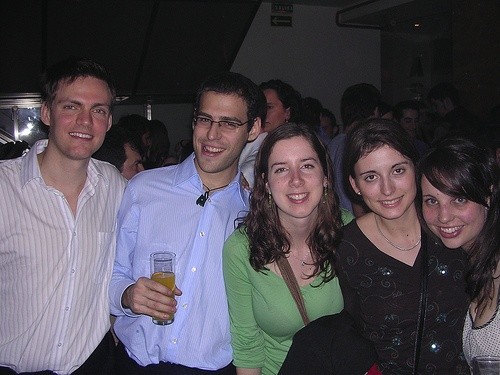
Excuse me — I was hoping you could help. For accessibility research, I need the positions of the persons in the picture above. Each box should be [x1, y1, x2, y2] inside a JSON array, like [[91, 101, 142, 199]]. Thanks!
[[0, 58, 128, 375], [331, 119, 475, 375], [260, 80, 301, 132], [222, 121, 356, 375], [107, 72, 268, 375], [415, 136, 500, 375], [92, 113, 193, 181], [300, 83, 500, 160]]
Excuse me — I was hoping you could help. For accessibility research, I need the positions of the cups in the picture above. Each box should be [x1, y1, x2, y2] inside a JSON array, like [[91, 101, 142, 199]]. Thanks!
[[473, 355, 500, 375], [150, 251, 176, 325]]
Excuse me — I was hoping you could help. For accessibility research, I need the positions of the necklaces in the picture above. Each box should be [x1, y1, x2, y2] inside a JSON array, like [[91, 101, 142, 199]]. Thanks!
[[282, 241, 310, 266], [196, 183, 228, 208], [375, 215, 421, 251]]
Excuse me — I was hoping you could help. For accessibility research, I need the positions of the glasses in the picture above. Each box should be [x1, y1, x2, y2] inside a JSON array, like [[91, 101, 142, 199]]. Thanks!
[[193, 116, 249, 132]]
